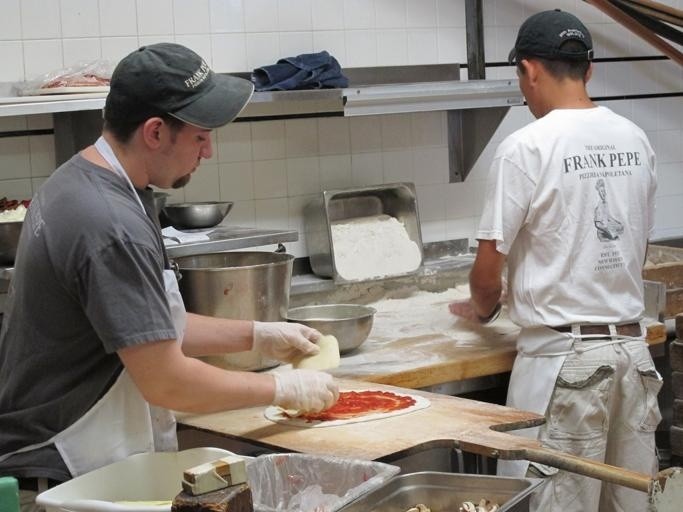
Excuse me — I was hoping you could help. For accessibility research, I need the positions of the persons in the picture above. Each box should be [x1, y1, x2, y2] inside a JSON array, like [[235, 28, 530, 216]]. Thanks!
[[447, 8, 666, 510], [0, 42, 340, 495]]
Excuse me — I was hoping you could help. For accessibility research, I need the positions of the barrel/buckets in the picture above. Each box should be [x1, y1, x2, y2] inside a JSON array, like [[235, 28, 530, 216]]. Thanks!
[[167, 242, 294, 372]]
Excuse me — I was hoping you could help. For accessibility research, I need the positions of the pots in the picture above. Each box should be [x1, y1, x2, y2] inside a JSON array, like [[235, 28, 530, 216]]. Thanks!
[[164, 243, 296, 375]]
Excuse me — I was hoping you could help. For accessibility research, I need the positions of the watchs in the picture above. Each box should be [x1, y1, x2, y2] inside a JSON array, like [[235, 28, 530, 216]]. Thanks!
[[475, 303, 503, 324]]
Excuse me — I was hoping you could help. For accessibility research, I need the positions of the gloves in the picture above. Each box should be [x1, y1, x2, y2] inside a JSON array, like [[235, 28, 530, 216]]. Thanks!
[[255, 320, 321, 361], [272, 370, 339, 412]]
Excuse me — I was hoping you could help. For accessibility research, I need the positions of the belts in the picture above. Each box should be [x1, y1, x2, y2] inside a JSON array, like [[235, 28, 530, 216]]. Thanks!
[[549, 322, 643, 339]]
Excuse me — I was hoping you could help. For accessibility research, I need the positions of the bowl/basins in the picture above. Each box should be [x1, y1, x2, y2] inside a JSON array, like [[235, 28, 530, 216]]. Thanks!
[[288, 304, 376, 355], [335, 470, 546, 512], [161, 201, 233, 227], [34, 446, 254, 511], [247, 453, 401, 512], [307, 185, 427, 280]]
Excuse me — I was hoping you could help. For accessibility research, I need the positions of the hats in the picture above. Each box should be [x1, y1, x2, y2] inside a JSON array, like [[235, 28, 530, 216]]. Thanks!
[[509, 8, 594, 61], [104, 43, 253, 130]]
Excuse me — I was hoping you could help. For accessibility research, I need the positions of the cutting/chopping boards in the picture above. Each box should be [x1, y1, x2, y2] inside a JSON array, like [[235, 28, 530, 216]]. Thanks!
[[330, 293, 664, 391], [174, 378, 652, 493]]
